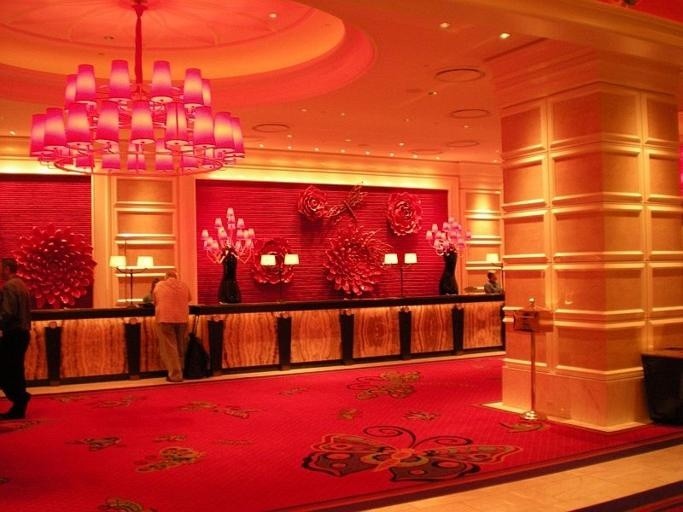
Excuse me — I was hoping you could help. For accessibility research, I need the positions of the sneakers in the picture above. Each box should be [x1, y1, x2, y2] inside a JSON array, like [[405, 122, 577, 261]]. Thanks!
[[0, 391, 31, 420]]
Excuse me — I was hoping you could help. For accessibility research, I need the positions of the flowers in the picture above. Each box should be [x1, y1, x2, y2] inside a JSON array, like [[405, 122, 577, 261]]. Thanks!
[[250, 183, 424, 297], [11, 223, 98, 309]]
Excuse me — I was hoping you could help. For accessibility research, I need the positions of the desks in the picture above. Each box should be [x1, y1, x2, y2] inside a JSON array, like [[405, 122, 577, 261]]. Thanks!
[[1, 299, 512, 384]]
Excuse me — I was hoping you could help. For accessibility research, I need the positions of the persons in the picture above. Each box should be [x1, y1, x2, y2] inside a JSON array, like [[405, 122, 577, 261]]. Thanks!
[[483, 270, 502, 294], [151, 270, 192, 384], [0, 257, 32, 420], [143, 280, 156, 302]]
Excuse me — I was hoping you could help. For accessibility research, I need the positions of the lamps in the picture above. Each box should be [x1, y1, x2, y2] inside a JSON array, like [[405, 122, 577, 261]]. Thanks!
[[110, 249, 156, 304], [28, 0, 248, 176], [201, 210, 302, 305]]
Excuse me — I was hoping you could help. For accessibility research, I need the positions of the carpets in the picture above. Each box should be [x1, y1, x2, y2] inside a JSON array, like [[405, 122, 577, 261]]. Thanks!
[[1, 347, 682, 512]]
